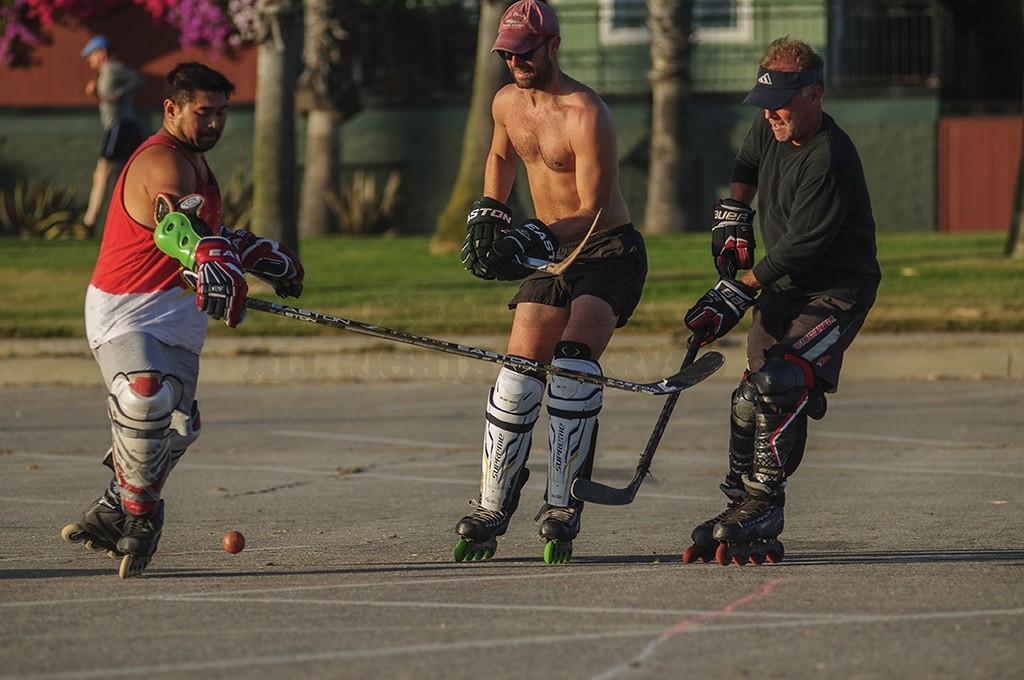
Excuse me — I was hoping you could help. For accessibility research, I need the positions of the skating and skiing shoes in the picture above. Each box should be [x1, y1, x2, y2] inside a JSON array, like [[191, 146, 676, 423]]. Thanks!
[[679, 477, 748, 563], [533, 503, 581, 563], [60, 489, 133, 560], [451, 496, 511, 560], [714, 472, 788, 568], [116, 498, 167, 579]]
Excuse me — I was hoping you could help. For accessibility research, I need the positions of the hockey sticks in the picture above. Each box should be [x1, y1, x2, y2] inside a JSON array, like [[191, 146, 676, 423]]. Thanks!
[[571, 329, 709, 506], [510, 208, 604, 276], [179, 267, 726, 396]]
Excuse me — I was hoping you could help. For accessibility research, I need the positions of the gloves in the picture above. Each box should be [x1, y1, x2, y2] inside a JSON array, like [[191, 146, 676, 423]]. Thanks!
[[222, 227, 302, 298], [192, 237, 248, 326], [492, 218, 556, 280], [460, 197, 512, 280], [712, 198, 756, 278], [685, 275, 757, 349]]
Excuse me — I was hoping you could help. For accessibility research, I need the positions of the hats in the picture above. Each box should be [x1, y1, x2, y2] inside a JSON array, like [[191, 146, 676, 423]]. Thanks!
[[493, 0, 558, 55], [742, 59, 821, 112], [80, 36, 106, 58]]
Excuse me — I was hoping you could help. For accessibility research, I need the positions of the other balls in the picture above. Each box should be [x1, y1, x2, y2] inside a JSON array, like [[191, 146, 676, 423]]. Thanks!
[[223, 531, 245, 554]]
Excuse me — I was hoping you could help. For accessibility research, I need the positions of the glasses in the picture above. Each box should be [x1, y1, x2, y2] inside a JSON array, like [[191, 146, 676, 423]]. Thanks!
[[496, 42, 550, 62]]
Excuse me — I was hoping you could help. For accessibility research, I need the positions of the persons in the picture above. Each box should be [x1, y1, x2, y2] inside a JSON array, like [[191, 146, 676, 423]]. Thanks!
[[684, 39, 882, 544], [80, 36, 143, 226], [80, 58, 306, 552], [456, 0, 648, 539]]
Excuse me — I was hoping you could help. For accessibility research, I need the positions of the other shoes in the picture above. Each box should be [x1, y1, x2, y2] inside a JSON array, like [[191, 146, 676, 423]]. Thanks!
[[61, 225, 93, 239]]
